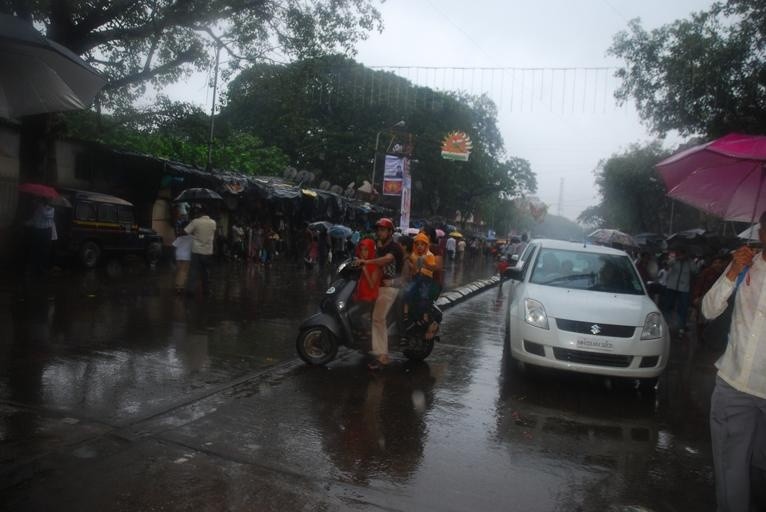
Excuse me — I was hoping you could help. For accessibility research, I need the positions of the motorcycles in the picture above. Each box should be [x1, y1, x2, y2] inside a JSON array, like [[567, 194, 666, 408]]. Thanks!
[[296, 240, 442, 366]]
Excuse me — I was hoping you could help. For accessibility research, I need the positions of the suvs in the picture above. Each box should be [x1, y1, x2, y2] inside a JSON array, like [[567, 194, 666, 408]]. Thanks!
[[54, 187, 164, 271]]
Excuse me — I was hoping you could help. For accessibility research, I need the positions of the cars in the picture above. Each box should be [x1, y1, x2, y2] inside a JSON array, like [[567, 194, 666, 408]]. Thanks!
[[504, 238, 670, 396]]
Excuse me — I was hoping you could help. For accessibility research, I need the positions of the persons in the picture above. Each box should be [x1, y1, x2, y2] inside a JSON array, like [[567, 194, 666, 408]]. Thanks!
[[396, 166, 403, 178], [624, 245, 738, 341], [701, 213, 766, 511], [168, 208, 280, 294], [352, 217, 438, 370], [305, 216, 527, 261], [27, 200, 58, 275]]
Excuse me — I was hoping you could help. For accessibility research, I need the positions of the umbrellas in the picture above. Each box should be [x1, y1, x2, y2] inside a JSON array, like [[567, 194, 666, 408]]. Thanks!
[[17, 180, 62, 201], [654, 133, 766, 247], [588, 223, 760, 252]]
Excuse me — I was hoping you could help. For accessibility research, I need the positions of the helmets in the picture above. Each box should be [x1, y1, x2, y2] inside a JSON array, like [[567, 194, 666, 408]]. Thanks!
[[376, 218, 393, 229]]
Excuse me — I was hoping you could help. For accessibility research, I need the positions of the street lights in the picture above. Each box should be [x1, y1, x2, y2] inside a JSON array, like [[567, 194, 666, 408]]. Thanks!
[[206, 62, 218, 164], [371, 121, 406, 188]]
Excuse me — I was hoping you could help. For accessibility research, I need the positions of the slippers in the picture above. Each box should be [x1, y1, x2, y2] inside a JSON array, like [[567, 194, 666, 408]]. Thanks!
[[426, 322, 439, 339], [370, 360, 393, 370]]
